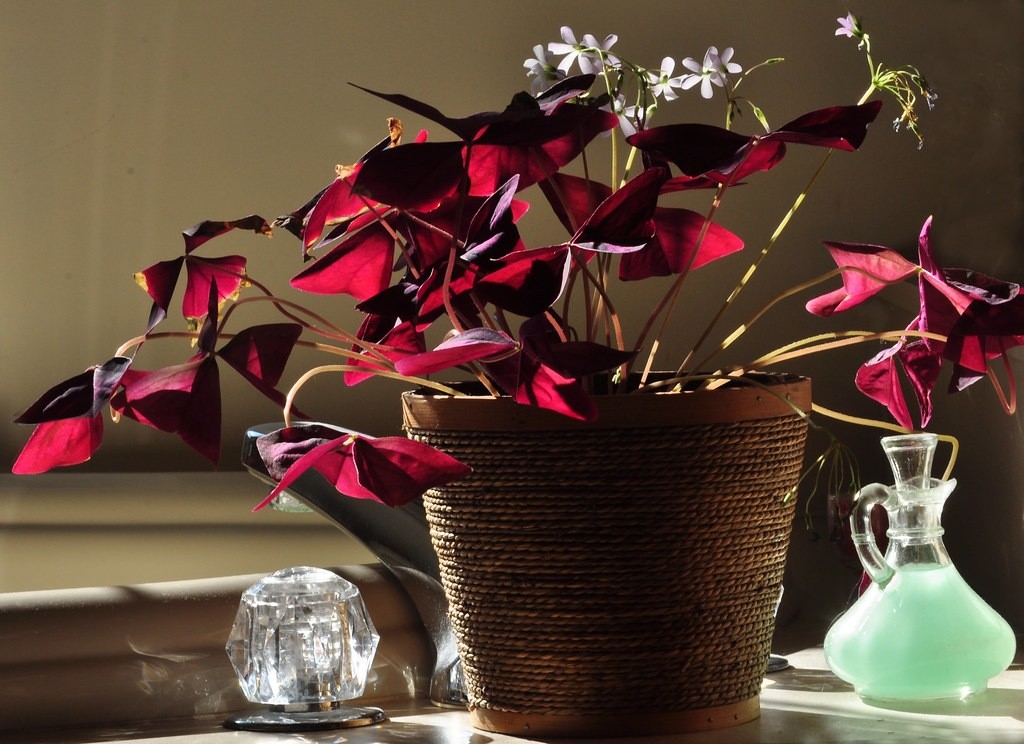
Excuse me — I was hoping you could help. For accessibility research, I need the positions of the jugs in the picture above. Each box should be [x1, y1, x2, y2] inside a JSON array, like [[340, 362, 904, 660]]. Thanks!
[[821, 433, 1017, 701]]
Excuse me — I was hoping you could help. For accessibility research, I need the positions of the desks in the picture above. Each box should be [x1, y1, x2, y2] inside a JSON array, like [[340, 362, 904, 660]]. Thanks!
[[83, 642, 1024, 744]]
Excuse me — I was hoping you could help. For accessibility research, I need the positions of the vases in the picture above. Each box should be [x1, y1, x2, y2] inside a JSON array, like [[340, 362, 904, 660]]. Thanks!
[[826, 431, 1015, 708]]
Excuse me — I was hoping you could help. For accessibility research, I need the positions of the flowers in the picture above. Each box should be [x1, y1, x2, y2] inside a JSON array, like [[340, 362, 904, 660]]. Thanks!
[[398, 369, 810, 737], [14, 10, 1023, 515]]
